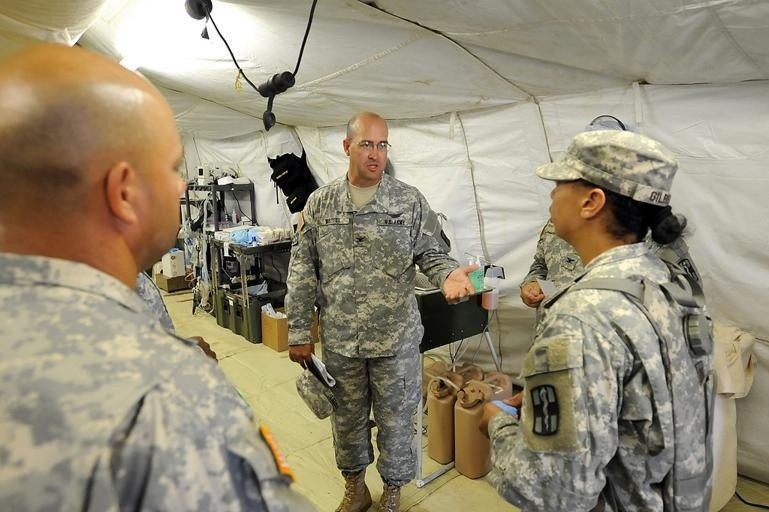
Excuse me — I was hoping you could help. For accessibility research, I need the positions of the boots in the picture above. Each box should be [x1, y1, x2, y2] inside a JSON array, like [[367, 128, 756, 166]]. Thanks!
[[335, 469, 372, 512], [377, 483, 401, 512]]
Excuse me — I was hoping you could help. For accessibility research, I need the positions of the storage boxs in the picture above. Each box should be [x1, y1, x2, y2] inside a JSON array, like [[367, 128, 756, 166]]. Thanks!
[[155, 273, 188, 292]]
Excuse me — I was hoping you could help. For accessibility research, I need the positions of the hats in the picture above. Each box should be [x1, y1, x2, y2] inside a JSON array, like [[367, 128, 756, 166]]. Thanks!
[[297, 358, 339, 419], [536, 129, 678, 207]]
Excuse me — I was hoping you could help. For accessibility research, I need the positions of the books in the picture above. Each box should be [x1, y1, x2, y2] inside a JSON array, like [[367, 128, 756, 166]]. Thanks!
[[304, 350, 336, 388]]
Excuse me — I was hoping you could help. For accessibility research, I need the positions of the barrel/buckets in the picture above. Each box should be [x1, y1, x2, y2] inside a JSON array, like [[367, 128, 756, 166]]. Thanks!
[[427, 362, 485, 465], [454, 373, 514, 480]]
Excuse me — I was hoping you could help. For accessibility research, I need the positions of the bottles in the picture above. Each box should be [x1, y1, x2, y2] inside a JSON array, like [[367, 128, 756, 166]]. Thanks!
[[198, 169, 205, 186]]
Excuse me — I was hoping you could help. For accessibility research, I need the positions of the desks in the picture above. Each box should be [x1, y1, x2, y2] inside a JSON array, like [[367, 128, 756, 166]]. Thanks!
[[198, 239, 292, 344]]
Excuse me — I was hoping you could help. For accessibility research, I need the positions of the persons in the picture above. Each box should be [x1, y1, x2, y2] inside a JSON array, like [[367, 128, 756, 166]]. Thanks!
[[476, 130, 714, 511], [284, 112, 480, 512], [520, 122, 701, 330], [134, 265, 220, 363], [0, 43, 318, 512]]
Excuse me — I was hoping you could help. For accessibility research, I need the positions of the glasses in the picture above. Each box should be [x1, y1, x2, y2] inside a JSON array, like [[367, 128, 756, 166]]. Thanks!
[[360, 142, 391, 152]]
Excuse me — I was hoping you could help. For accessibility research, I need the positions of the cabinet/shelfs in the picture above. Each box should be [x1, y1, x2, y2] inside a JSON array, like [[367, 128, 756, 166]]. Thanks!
[[185, 185, 255, 318]]
